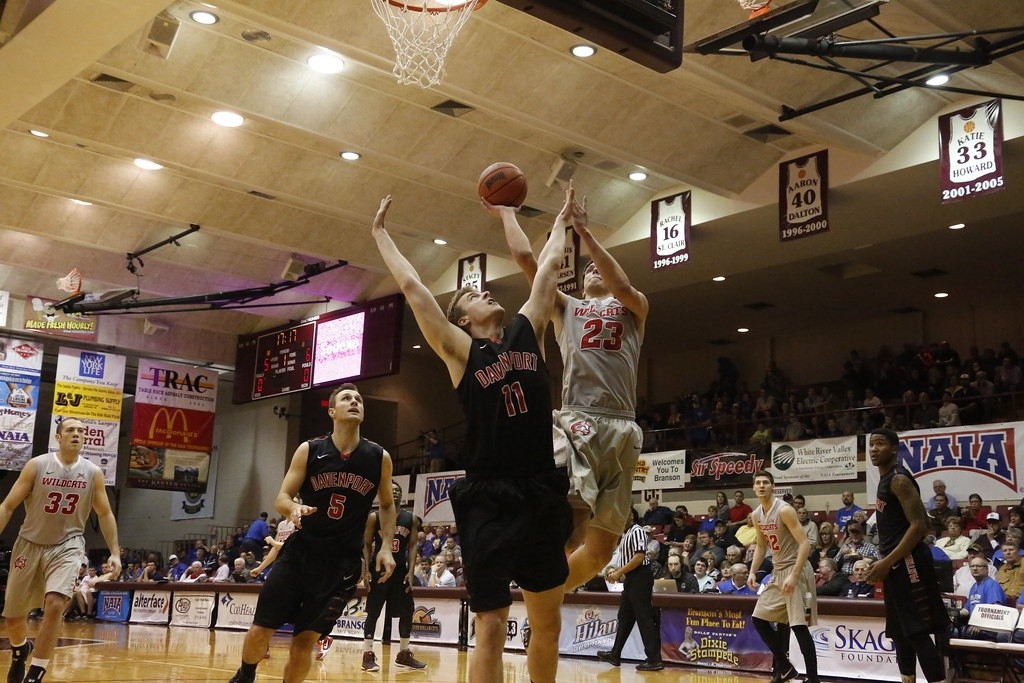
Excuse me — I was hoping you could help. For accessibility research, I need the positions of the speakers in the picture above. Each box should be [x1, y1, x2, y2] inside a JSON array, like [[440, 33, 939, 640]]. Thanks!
[[545, 156, 579, 191]]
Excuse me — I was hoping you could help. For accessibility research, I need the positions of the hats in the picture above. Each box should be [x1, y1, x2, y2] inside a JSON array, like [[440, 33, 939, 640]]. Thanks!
[[1006, 528, 1024, 540], [643, 525, 653, 533], [169, 554, 178, 560], [261, 512, 268, 517], [783, 494, 793, 499], [986, 512, 1001, 520], [715, 519, 726, 524], [966, 544, 984, 551], [247, 551, 255, 557], [850, 523, 862, 532]]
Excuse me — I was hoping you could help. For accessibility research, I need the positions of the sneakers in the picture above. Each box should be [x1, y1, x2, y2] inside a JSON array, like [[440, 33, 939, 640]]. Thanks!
[[23, 665, 46, 683], [7, 640, 34, 683], [769, 666, 799, 683], [803, 677, 819, 683], [265, 644, 270, 657], [361, 651, 380, 671], [316, 635, 334, 659], [395, 648, 427, 669], [636, 660, 662, 670], [597, 651, 621, 666]]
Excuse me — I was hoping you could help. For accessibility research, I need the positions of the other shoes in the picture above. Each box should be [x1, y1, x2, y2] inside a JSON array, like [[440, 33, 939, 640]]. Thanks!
[[229, 668, 255, 683]]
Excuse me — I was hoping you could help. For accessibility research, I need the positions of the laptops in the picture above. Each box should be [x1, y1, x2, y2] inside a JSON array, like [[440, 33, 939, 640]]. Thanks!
[[653, 579, 678, 593]]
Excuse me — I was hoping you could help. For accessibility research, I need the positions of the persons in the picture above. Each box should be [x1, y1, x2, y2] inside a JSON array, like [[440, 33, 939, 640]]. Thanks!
[[362, 482, 428, 672], [0, 416, 122, 683], [356, 522, 466, 588], [249, 497, 333, 660], [424, 429, 445, 473], [635, 340, 1023, 460], [479, 195, 649, 595], [229, 383, 397, 683], [597, 508, 664, 672], [744, 469, 820, 683], [370, 178, 577, 683], [65, 517, 277, 620], [572, 480, 1024, 644], [861, 428, 952, 683]]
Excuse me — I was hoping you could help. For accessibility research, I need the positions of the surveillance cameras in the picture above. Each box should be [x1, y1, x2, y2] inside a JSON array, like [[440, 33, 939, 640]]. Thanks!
[[51, 292, 87, 310]]
